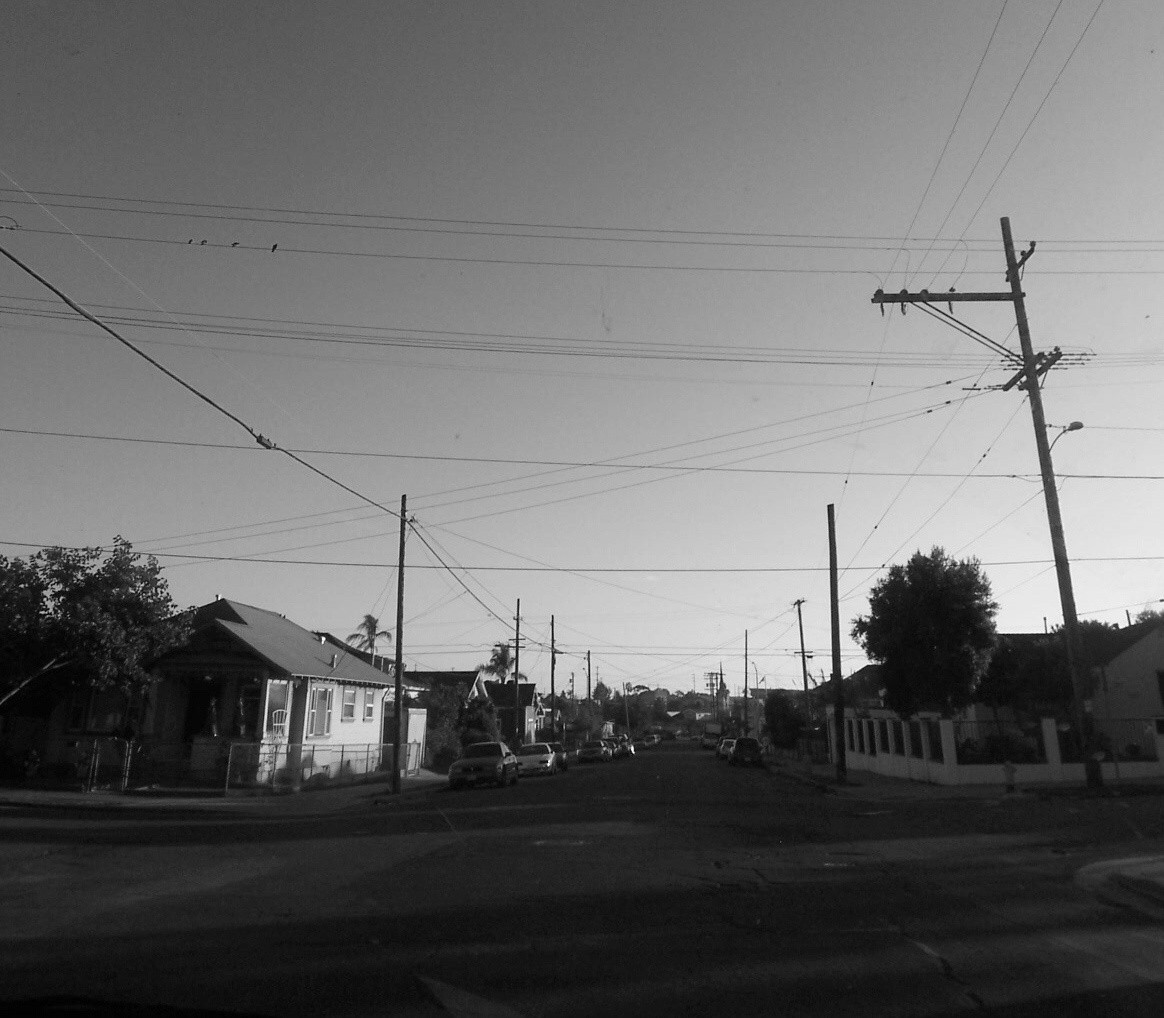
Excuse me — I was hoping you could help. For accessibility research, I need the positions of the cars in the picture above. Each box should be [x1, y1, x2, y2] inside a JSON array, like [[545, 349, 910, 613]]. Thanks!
[[727, 738, 765, 767], [448, 742, 520, 791], [577, 734, 637, 763], [515, 743, 560, 777], [633, 732, 663, 750], [546, 741, 569, 772], [700, 733, 736, 760]]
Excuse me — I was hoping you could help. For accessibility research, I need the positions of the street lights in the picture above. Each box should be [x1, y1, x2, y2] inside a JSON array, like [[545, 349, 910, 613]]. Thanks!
[[1039, 421, 1110, 797]]
